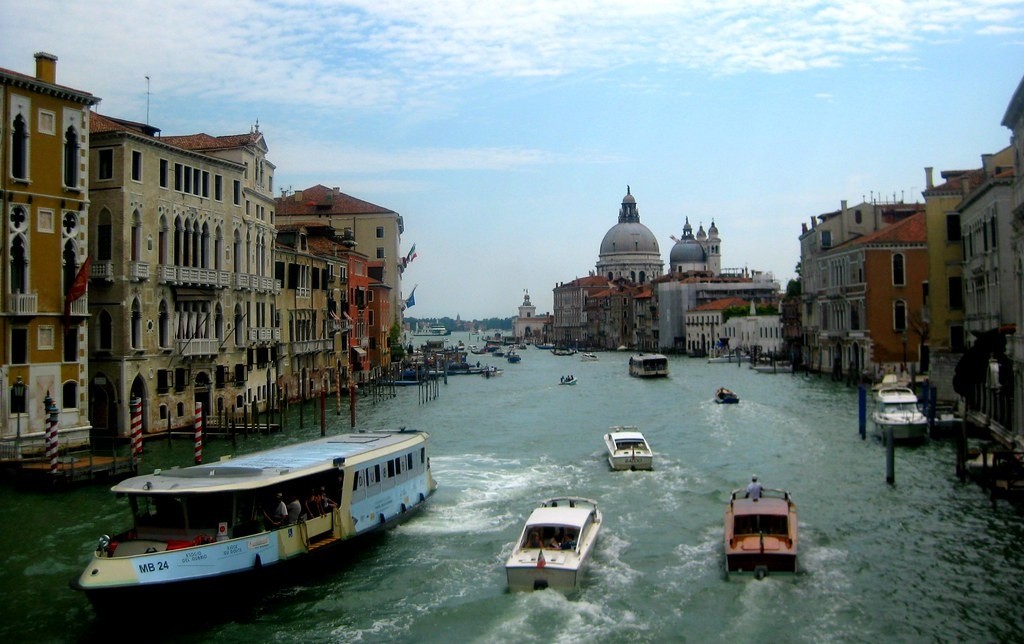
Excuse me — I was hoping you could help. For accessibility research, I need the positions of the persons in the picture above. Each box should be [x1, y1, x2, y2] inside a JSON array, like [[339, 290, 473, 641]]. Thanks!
[[476, 361, 480, 367], [264, 484, 338, 525], [879, 362, 905, 372], [561, 375, 574, 382], [526, 527, 576, 549], [744, 475, 763, 498], [718, 388, 726, 400], [491, 366, 494, 371], [403, 357, 417, 370]]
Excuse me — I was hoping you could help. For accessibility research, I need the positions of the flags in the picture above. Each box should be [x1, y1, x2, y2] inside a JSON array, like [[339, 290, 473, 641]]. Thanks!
[[538, 552, 546, 572], [406, 244, 417, 261], [65, 257, 89, 303], [406, 286, 415, 308]]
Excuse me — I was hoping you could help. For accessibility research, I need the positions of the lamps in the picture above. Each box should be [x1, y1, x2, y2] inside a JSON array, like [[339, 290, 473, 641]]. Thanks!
[[153, 467, 161, 476], [143, 482, 152, 490], [332, 456, 346, 467]]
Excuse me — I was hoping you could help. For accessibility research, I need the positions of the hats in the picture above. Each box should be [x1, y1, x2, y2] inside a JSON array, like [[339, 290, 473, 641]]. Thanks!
[[276, 493, 283, 498]]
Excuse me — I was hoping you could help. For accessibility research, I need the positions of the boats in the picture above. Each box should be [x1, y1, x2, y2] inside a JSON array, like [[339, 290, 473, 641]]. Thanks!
[[603, 424, 654, 472], [627, 352, 671, 379], [67, 425, 440, 632], [752, 364, 793, 374], [707, 353, 751, 363], [714, 386, 740, 405], [580, 352, 598, 362], [558, 374, 577, 385], [617, 345, 628, 352], [724, 486, 800, 581], [399, 323, 579, 376], [504, 493, 603, 596], [870, 383, 928, 447]]
